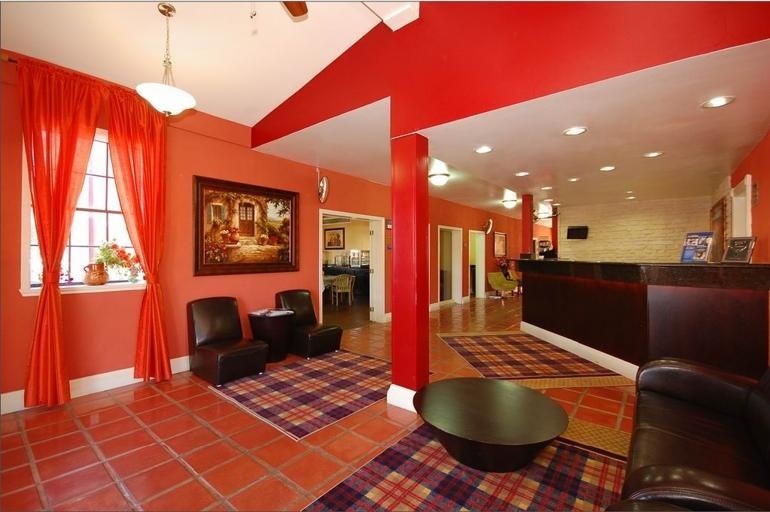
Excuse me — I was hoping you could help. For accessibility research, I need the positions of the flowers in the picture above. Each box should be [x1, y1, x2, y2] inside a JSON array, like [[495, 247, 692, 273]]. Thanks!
[[93, 240, 147, 277]]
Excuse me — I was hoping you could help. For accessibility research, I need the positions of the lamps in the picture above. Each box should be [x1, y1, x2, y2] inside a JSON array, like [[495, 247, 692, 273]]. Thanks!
[[502, 190, 517, 209], [482, 218, 494, 235], [429, 162, 452, 186], [316, 168, 329, 204], [133, 2, 196, 117]]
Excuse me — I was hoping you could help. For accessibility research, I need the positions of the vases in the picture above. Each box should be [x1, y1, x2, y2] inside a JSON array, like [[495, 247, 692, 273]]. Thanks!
[[127, 273, 144, 284], [82, 262, 109, 288]]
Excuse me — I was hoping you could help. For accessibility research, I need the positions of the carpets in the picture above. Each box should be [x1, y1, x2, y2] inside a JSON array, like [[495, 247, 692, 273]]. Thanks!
[[436, 330, 635, 388], [299, 416, 627, 512], [208, 346, 437, 443]]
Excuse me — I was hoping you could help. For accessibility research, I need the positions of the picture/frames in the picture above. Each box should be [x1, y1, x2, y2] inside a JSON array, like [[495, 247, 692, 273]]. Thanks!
[[681, 231, 714, 262], [721, 237, 756, 264], [191, 174, 300, 277], [493, 232, 508, 258], [324, 227, 345, 250]]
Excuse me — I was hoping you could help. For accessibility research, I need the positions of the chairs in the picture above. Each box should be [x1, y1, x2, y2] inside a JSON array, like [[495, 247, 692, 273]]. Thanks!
[[330, 273, 355, 307], [488, 271, 515, 299]]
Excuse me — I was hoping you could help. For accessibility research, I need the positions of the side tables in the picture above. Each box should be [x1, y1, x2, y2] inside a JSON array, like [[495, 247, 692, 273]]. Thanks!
[[248, 307, 294, 360]]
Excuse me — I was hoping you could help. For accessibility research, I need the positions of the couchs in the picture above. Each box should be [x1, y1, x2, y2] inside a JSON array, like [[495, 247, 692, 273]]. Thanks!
[[274, 288, 343, 361], [187, 296, 268, 388], [604, 355, 770, 512]]
